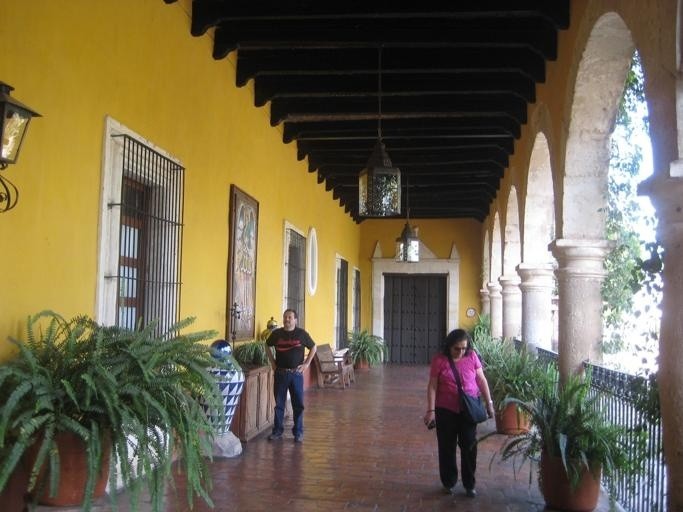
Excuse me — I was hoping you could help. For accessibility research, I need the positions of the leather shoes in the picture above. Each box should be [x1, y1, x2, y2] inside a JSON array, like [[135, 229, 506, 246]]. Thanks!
[[269, 434, 281, 440], [444, 486, 454, 494], [466, 489, 476, 497], [294, 436, 303, 441]]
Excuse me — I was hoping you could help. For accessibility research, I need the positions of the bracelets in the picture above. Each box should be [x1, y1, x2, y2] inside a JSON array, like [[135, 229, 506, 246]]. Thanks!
[[427, 409, 435, 414], [487, 400, 493, 408]]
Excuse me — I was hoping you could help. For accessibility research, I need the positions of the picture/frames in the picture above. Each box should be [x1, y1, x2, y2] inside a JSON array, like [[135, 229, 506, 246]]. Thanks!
[[225, 184, 256, 342]]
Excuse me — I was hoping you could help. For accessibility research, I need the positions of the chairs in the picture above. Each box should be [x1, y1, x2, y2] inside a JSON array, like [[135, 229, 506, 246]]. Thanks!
[[313, 343, 356, 389]]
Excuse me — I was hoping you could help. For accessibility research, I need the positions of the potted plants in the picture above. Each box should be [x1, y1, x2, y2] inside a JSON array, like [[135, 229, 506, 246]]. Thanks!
[[488, 362, 650, 511], [484, 352, 560, 434], [347, 326, 390, 369], [0, 310, 224, 511]]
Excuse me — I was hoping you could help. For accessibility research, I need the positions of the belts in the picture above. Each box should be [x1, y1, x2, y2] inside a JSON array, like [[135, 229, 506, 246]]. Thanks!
[[279, 368, 295, 372]]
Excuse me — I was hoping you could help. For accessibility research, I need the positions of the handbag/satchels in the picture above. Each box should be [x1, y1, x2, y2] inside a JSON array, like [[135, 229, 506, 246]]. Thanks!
[[461, 395, 491, 423]]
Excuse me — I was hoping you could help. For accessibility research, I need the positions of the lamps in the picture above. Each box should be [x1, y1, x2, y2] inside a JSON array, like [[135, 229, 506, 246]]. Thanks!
[[397, 167, 422, 263], [0, 83, 41, 211], [359, 47, 401, 217]]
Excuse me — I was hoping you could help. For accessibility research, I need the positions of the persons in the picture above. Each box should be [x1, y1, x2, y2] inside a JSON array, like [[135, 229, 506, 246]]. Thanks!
[[423, 329, 496, 497], [265, 309, 317, 443]]
